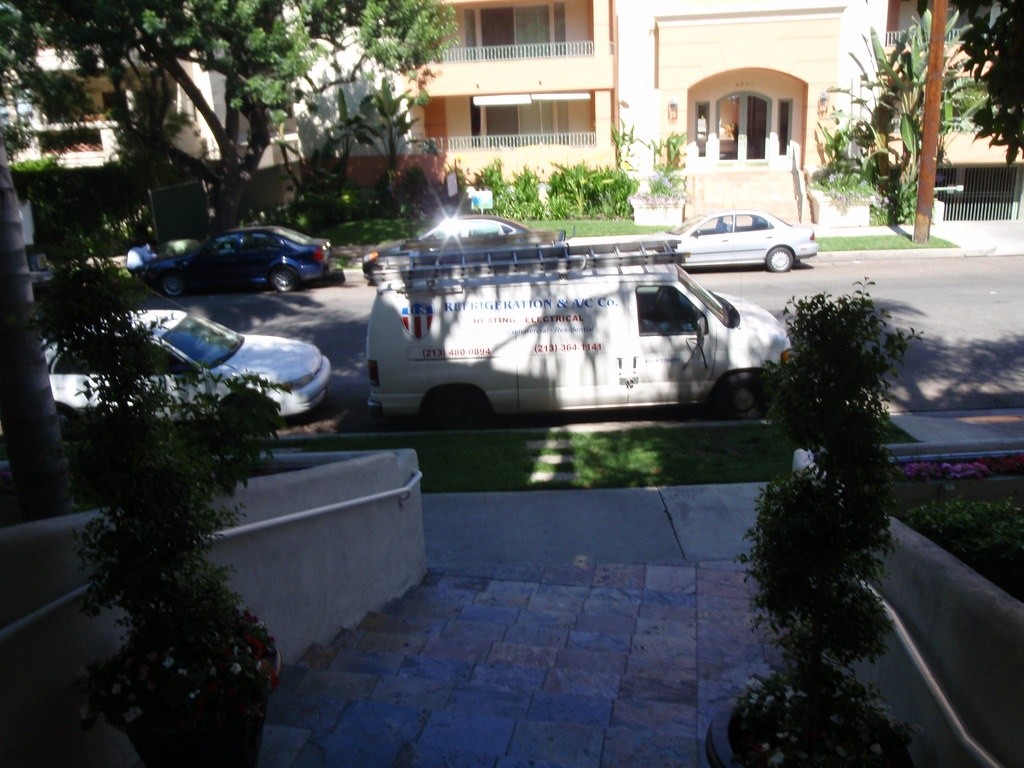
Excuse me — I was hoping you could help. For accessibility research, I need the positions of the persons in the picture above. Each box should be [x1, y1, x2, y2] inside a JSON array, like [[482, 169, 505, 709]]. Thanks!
[[127, 240, 161, 276]]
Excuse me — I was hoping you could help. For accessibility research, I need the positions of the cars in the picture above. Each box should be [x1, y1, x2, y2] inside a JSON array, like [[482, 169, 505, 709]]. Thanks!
[[652, 208, 821, 273], [362, 213, 556, 285], [40, 306, 334, 417], [135, 225, 332, 300]]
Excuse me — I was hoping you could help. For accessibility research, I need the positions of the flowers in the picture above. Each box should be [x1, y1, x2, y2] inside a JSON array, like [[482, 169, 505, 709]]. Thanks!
[[80, 608, 281, 732], [737, 674, 924, 768]]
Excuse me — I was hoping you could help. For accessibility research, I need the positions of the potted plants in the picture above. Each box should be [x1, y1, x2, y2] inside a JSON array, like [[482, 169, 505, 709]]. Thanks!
[[29, 214, 291, 768], [704, 277, 926, 768]]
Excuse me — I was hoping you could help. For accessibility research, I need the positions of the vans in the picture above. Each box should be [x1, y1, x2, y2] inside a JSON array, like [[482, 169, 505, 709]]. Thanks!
[[364, 235, 792, 428]]
